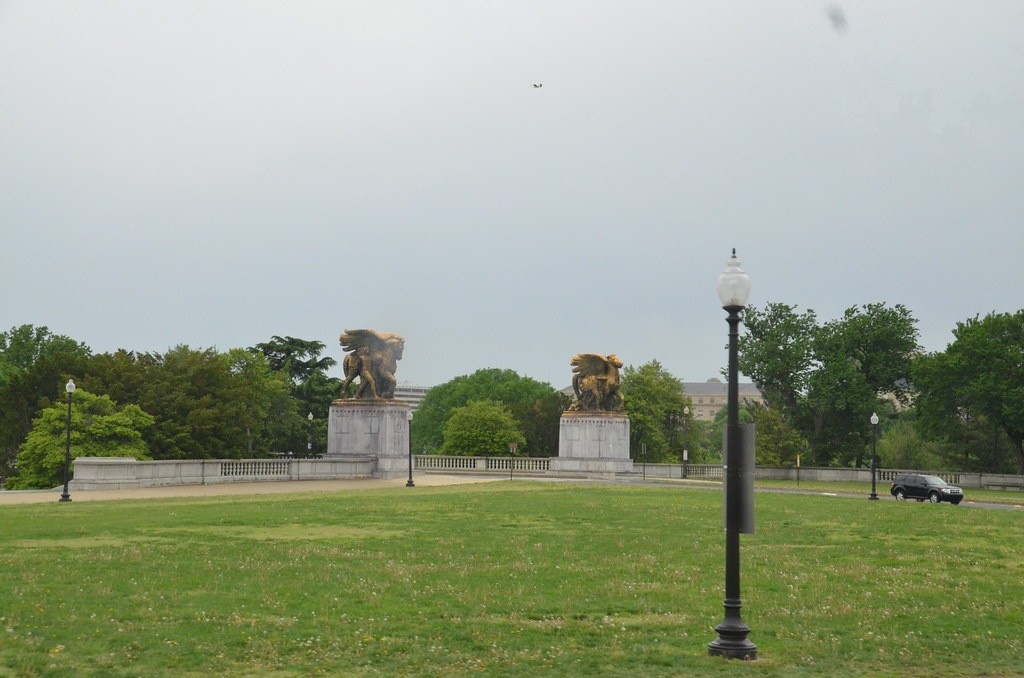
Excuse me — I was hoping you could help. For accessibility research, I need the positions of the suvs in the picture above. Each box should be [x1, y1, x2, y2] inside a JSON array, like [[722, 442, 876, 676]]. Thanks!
[[890, 473, 964, 505]]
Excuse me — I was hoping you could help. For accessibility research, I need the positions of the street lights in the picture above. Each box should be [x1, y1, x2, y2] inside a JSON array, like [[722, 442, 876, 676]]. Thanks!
[[406, 408, 415, 487], [307, 412, 313, 458], [868, 412, 880, 501], [711, 245, 760, 662], [681, 406, 690, 479], [57, 377, 76, 502]]
